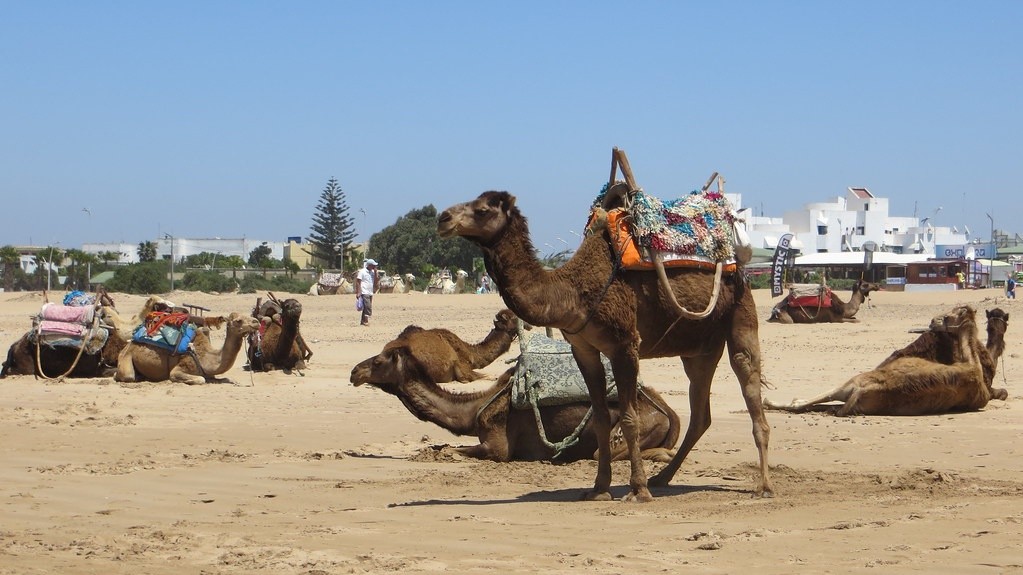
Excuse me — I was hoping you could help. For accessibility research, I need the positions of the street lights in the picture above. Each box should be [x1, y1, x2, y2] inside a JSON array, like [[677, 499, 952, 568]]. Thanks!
[[986, 213, 994, 287], [47, 241, 61, 292], [164, 232, 174, 291]]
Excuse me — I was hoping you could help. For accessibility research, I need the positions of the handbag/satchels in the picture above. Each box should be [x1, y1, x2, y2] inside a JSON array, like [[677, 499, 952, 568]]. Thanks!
[[357, 296, 363, 311]]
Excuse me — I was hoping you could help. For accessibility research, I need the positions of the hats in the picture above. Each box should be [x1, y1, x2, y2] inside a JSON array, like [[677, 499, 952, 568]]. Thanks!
[[367, 259, 378, 265]]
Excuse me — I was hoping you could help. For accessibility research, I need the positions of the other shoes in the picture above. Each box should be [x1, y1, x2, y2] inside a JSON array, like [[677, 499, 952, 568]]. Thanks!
[[362, 320, 369, 326]]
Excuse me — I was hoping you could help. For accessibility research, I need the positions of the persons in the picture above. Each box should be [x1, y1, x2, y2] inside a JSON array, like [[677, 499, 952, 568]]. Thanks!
[[357, 258, 379, 327], [1006, 274, 1015, 299], [955, 268, 964, 289], [785, 268, 809, 285], [477, 271, 492, 294]]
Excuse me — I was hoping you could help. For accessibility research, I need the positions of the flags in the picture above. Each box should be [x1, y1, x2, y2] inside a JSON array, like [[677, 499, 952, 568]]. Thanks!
[[771, 234, 793, 298]]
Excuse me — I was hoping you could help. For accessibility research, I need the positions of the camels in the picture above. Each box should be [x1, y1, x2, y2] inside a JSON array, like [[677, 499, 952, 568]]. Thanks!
[[245, 296, 304, 373], [876, 309, 1009, 400], [439, 180, 773, 501], [764, 306, 990, 417], [767, 279, 879, 322], [351, 310, 682, 462], [0, 305, 144, 378], [112, 297, 261, 385]]
